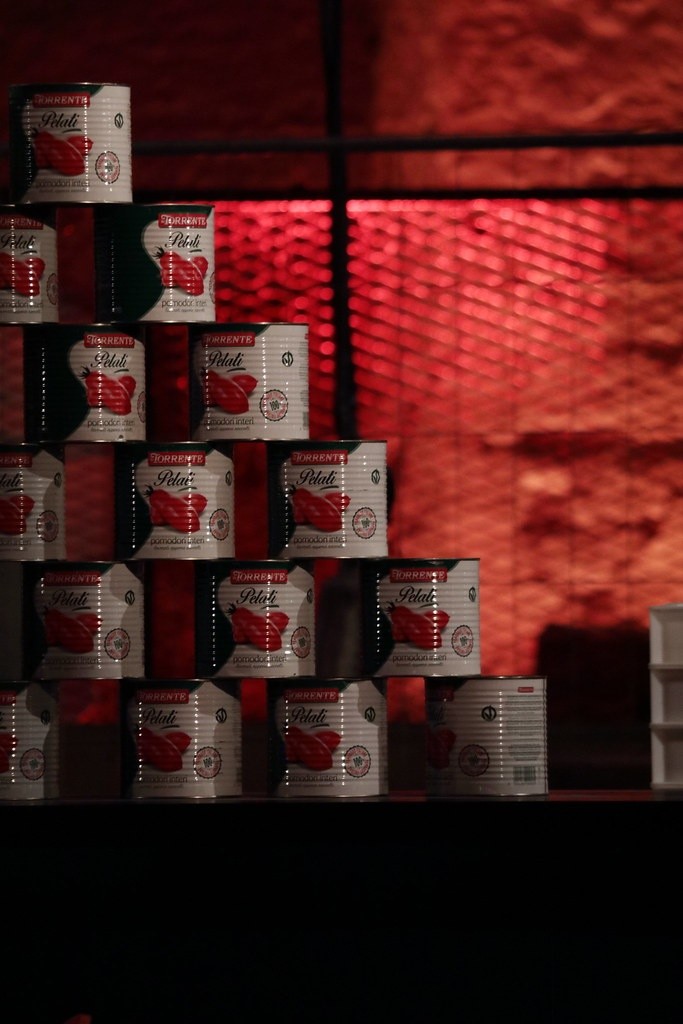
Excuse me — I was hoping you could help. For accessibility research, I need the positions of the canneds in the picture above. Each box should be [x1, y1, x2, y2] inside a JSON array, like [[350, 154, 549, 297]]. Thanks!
[[0, 322, 548, 806], [0, 80, 216, 324]]
